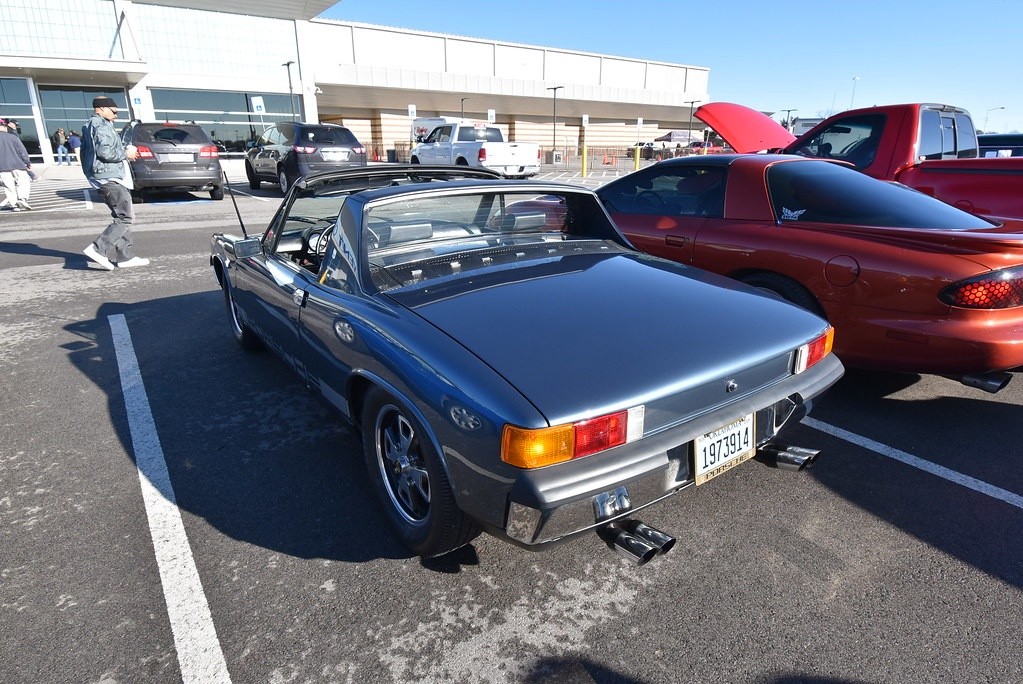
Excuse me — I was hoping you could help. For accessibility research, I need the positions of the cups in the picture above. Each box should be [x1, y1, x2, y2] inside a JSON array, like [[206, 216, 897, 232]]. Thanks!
[[126, 145, 137, 160]]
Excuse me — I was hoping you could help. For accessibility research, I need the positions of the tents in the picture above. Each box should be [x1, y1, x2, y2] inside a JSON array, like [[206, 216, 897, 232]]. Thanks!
[[652, 131, 700, 162]]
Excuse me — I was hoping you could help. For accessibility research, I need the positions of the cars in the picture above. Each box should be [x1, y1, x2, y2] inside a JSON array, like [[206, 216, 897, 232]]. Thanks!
[[491, 156, 1023, 395], [208, 166, 845, 565]]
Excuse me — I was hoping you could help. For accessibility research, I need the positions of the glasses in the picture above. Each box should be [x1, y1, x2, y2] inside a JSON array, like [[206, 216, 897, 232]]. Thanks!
[[107, 106, 117, 115]]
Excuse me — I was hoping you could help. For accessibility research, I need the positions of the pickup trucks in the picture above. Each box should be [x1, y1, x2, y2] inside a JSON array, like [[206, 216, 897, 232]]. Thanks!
[[409, 121, 542, 182], [683, 142, 725, 154], [690, 102, 1023, 236]]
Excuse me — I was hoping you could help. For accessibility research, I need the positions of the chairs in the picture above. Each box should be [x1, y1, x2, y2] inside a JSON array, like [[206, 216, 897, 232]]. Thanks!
[[502, 211, 546, 233], [379, 222, 433, 245]]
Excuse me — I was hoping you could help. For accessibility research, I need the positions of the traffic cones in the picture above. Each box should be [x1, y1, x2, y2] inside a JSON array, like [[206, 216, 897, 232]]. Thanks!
[[602, 154, 608, 165]]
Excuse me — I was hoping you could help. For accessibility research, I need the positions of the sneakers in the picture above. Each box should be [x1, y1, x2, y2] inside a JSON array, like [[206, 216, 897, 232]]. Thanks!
[[83, 243, 114, 271], [117, 256, 150, 267]]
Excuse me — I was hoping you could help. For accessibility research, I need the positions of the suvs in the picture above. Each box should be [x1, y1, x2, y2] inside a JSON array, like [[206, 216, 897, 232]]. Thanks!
[[244, 121, 367, 196], [627, 141, 659, 157], [120, 119, 226, 202]]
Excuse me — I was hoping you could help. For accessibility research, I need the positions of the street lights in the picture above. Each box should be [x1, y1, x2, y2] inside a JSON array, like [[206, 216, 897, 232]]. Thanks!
[[547, 86, 565, 150], [282, 60, 296, 122], [780, 108, 797, 128], [461, 97, 468, 118], [851, 76, 860, 106]]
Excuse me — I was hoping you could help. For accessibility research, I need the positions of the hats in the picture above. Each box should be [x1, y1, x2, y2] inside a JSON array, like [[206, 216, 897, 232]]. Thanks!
[[10, 119, 20, 126]]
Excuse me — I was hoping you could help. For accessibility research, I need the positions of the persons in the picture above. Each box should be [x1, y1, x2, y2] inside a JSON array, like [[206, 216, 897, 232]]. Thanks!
[[81, 95, 150, 271], [52, 127, 83, 166], [0, 118, 34, 212]]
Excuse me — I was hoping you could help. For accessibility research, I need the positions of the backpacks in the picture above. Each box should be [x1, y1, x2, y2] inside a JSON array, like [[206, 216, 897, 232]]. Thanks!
[[80, 120, 103, 190]]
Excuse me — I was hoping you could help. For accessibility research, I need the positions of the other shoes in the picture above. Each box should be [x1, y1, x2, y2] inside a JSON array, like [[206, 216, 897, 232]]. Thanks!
[[68, 162, 72, 166], [58, 163, 61, 165], [13, 207, 21, 211], [18, 199, 32, 210]]
